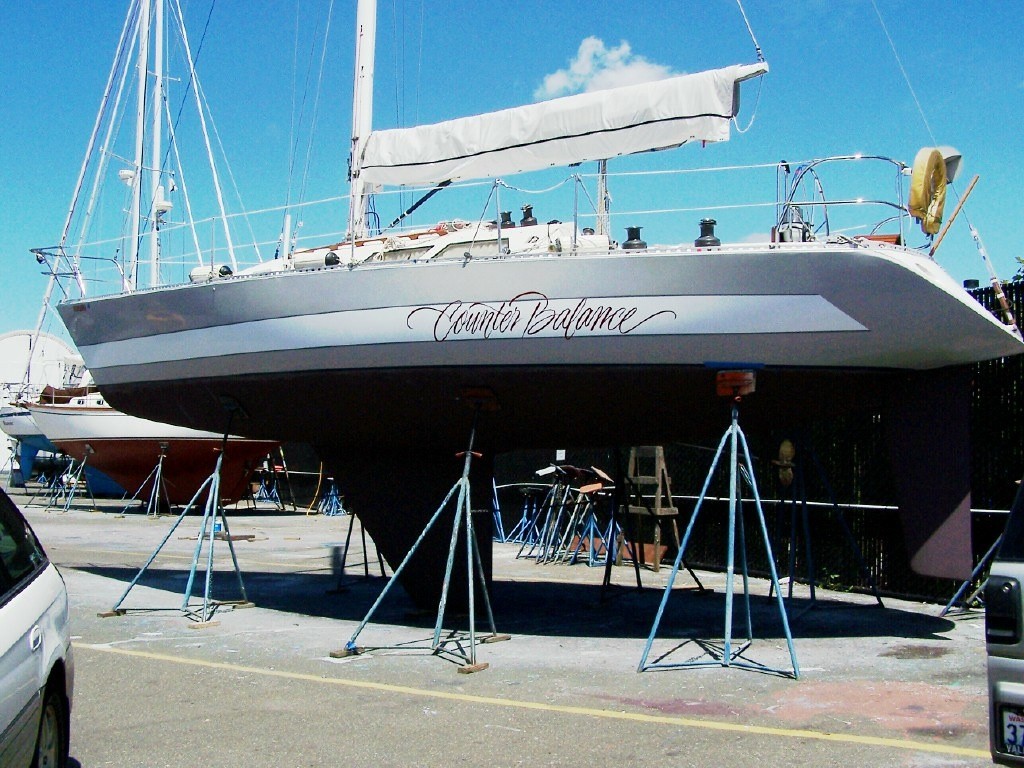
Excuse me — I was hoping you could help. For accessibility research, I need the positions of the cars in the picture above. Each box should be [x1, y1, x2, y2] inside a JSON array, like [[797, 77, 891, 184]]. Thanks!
[[980, 475, 1024, 768], [0, 484, 75, 768]]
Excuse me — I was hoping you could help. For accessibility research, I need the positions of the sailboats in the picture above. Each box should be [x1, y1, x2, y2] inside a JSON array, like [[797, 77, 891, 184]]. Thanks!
[[0, 2, 1024, 609]]
